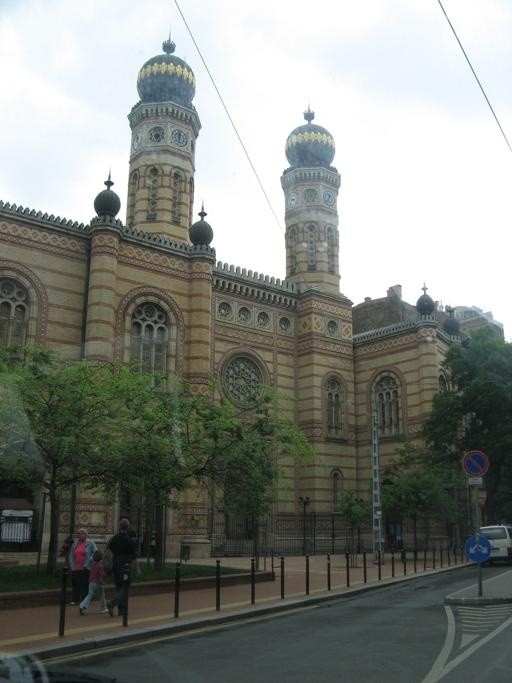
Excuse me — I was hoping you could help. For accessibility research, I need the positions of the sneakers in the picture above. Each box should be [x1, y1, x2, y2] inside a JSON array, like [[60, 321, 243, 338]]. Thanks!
[[70, 600, 121, 617]]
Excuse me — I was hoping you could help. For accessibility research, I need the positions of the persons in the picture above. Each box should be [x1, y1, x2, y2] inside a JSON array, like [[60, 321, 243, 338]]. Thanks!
[[104, 517, 137, 615], [69, 527, 97, 604], [78, 549, 109, 616]]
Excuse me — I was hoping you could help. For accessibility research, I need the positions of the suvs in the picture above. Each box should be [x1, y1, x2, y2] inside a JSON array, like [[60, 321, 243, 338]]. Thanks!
[[479, 522, 512, 567]]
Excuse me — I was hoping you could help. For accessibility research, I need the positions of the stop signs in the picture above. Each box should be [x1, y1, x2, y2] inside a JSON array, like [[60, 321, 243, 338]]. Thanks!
[[463, 450, 489, 477]]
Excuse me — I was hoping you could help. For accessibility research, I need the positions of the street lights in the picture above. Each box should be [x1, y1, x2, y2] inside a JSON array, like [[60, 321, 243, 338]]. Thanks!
[[298, 495, 311, 556]]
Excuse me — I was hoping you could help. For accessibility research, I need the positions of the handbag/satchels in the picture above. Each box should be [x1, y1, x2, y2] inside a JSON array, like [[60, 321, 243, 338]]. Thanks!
[[103, 550, 113, 569]]
